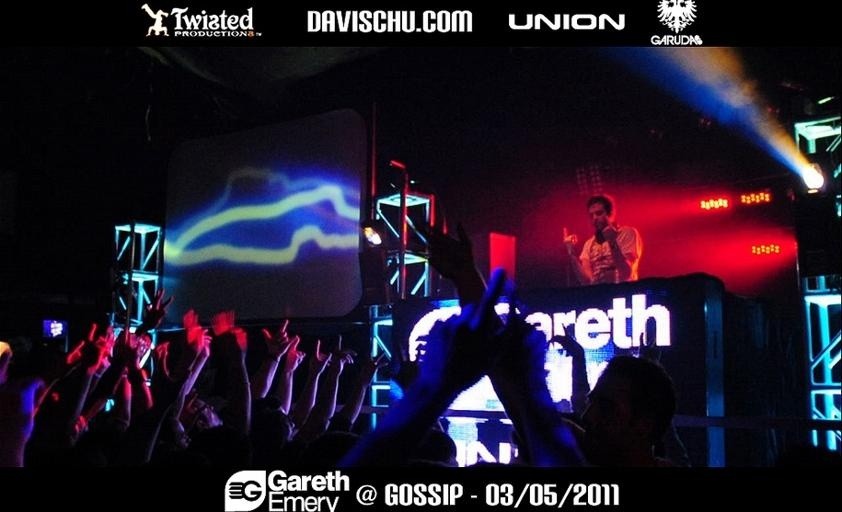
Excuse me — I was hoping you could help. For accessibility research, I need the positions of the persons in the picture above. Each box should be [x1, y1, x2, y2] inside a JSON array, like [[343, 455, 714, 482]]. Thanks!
[[562, 191, 643, 286]]
